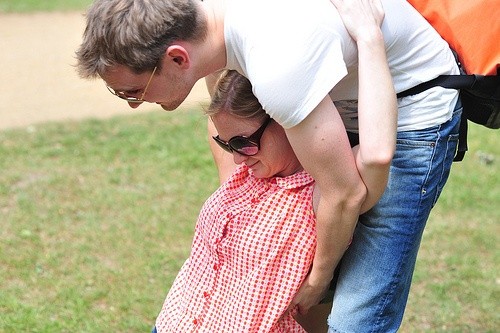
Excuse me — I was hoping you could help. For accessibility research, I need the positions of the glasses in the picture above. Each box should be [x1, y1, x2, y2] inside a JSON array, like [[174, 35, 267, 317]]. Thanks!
[[105, 64, 159, 103], [212, 116, 274, 157]]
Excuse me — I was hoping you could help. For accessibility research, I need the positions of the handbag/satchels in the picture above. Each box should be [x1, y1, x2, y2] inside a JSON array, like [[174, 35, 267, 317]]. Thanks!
[[406, 1, 500, 131]]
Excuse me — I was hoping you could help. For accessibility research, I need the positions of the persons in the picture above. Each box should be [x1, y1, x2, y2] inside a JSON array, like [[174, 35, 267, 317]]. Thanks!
[[76, 0, 465, 332], [149, 0, 400, 332]]
